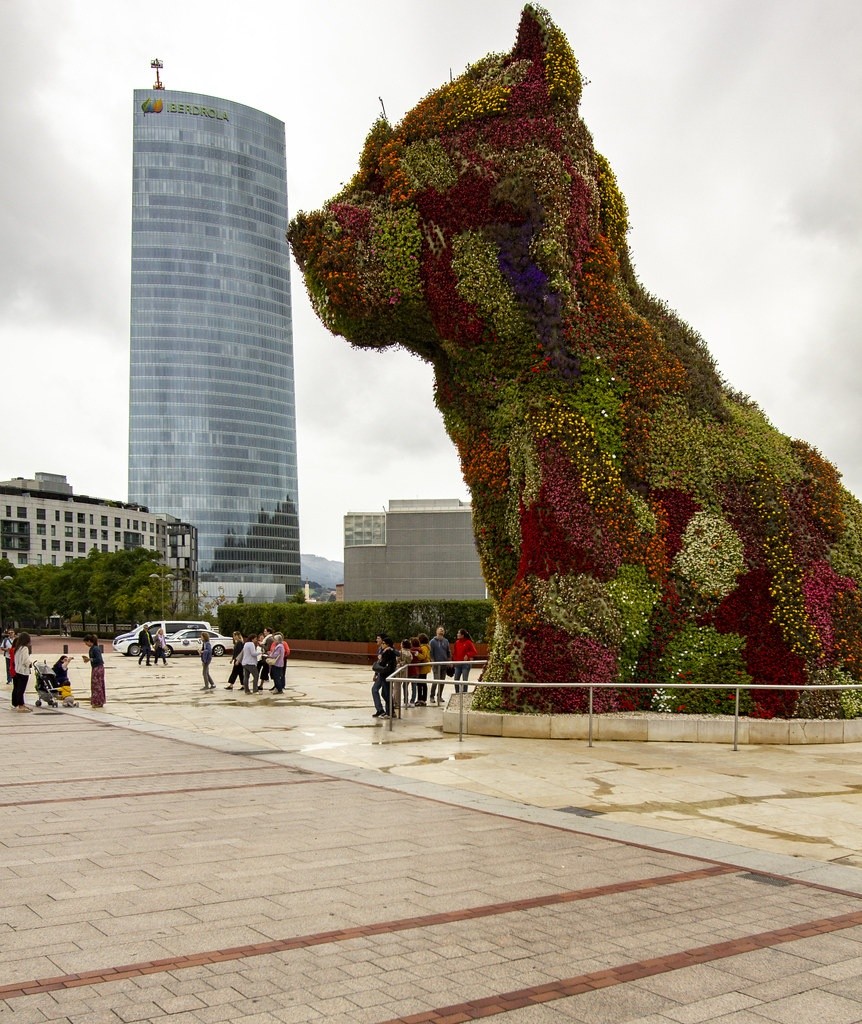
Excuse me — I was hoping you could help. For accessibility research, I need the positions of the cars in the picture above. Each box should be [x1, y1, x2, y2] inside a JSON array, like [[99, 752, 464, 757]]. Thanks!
[[149, 627, 238, 660]]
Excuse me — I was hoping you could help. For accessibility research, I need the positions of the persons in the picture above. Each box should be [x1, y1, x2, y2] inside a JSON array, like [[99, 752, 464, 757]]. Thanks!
[[9, 633, 33, 712], [371, 633, 432, 719], [199, 632, 217, 690], [57, 680, 79, 708], [52, 655, 73, 698], [138, 625, 154, 666], [82, 635, 106, 708], [451, 630, 478, 693], [1, 629, 17, 684], [223, 628, 290, 695], [152, 628, 169, 666], [430, 627, 451, 703]]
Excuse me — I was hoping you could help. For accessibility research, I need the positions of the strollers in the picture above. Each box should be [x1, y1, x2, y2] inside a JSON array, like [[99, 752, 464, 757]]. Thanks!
[[31, 659, 61, 709]]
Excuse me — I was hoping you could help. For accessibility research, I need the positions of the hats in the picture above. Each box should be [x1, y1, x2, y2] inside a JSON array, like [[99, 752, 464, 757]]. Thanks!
[[274, 632, 284, 640], [274, 635, 283, 643], [377, 633, 393, 646]]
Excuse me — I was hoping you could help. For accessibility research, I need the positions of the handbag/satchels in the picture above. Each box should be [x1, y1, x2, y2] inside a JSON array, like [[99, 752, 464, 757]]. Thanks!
[[265, 658, 276, 665], [447, 667, 455, 677], [165, 646, 168, 651], [372, 661, 385, 673]]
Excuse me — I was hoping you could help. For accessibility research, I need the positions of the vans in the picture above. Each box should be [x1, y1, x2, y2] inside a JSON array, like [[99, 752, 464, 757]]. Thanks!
[[112, 617, 216, 659]]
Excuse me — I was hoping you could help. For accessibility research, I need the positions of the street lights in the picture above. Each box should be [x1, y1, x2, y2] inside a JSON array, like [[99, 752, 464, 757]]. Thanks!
[[149, 558, 179, 621], [0, 576, 13, 644]]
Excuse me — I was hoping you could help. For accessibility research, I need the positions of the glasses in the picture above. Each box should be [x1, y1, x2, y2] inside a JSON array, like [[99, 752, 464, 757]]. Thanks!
[[9, 632, 14, 634], [382, 643, 387, 645]]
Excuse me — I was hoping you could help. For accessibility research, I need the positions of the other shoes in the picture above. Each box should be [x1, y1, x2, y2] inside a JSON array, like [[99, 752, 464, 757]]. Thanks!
[[11, 705, 31, 712], [138, 662, 151, 666], [271, 688, 282, 694], [6, 679, 13, 684], [67, 701, 79, 708], [378, 712, 397, 719], [200, 685, 216, 690], [394, 697, 445, 709], [269, 686, 276, 691], [223, 686, 264, 695], [372, 710, 385, 718], [155, 662, 168, 666]]
